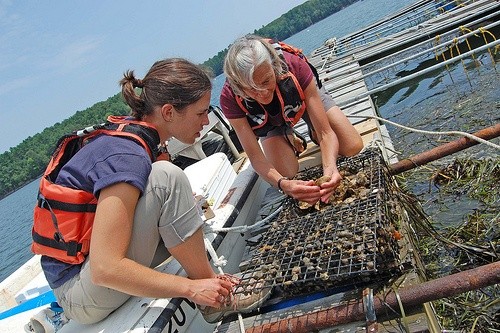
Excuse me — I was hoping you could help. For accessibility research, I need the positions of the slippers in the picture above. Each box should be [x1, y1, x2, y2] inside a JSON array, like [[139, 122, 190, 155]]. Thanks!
[[292, 129, 307, 152], [307, 124, 320, 146]]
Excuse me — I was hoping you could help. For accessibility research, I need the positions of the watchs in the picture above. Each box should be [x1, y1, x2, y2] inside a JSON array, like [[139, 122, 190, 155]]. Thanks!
[[278, 176, 289, 194]]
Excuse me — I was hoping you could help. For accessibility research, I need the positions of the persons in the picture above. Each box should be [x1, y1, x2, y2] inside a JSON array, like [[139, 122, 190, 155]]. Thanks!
[[31, 57, 274, 324], [219, 34, 363, 216]]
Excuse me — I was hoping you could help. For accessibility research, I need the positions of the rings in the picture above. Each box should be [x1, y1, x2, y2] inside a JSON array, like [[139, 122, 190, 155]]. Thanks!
[[220, 296, 224, 303]]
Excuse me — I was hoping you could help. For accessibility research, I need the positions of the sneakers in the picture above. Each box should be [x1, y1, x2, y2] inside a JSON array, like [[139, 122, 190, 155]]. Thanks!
[[196, 286, 273, 324]]
